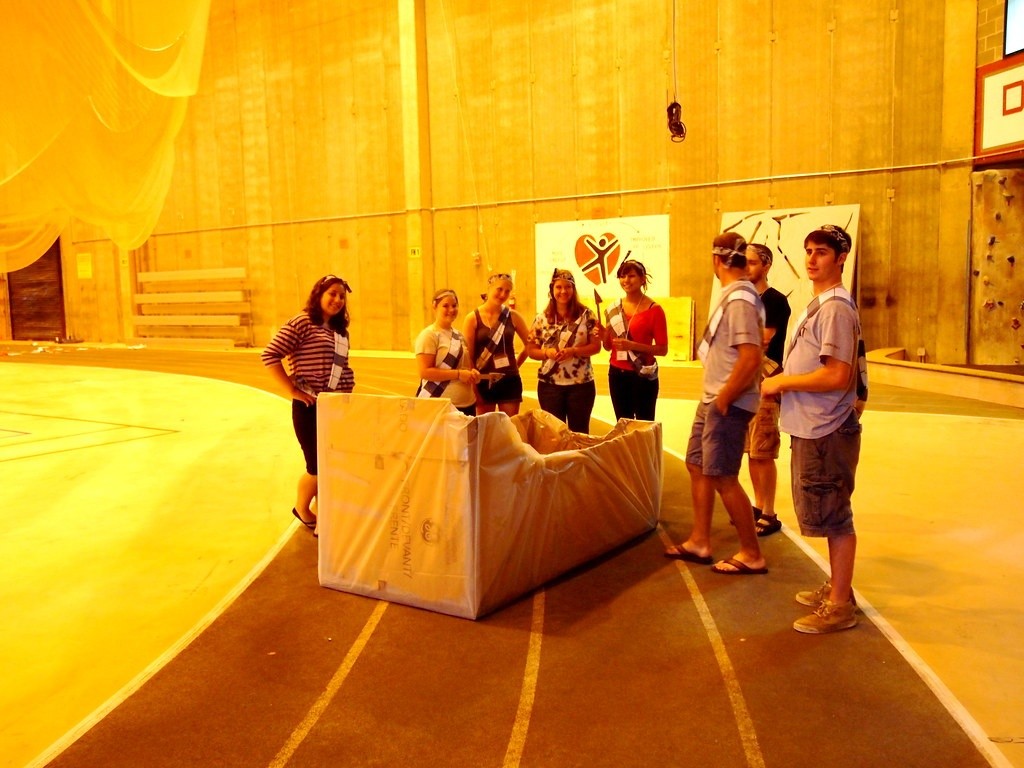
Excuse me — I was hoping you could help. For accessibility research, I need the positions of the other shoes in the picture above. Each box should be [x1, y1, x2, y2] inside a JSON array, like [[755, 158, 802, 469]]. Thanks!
[[795, 581, 856, 607], [794, 599, 857, 633]]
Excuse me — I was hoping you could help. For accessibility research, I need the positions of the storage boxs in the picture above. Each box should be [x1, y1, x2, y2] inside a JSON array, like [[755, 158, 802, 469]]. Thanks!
[[316, 391, 662, 620]]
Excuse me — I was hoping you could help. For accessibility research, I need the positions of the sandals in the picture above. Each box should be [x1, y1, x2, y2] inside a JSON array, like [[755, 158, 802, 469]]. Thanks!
[[730, 507, 764, 523], [756, 514, 781, 536]]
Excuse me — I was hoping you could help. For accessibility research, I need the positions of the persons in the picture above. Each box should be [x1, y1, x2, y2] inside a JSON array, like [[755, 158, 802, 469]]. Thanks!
[[464, 274, 529, 419], [667, 232, 769, 573], [261, 274, 355, 537], [595, 259, 669, 422], [759, 225, 867, 634], [413, 288, 481, 418], [523, 269, 601, 433], [729, 244, 792, 536]]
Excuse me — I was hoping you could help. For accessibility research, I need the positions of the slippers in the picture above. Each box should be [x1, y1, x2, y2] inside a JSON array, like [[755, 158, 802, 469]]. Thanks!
[[292, 507, 319, 539], [664, 543, 713, 565], [711, 555, 769, 574]]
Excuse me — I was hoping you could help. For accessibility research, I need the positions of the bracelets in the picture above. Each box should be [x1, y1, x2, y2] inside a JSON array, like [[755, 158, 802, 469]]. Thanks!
[[457, 369, 460, 380]]
